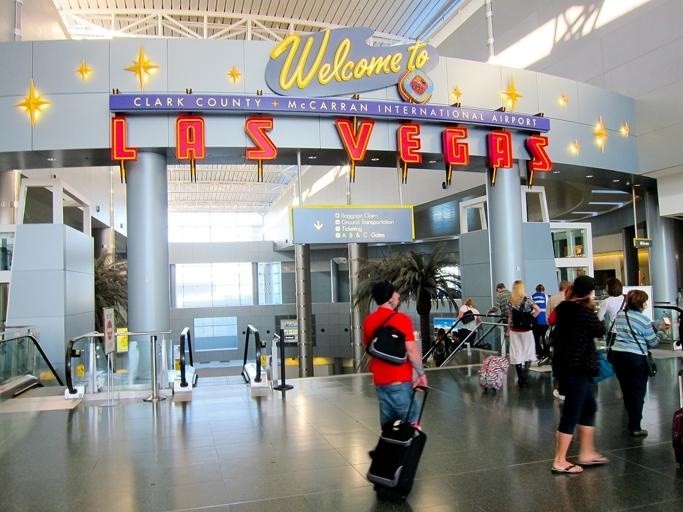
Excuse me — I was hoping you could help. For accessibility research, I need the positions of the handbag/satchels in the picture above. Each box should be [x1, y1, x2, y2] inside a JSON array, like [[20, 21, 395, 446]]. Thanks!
[[606, 332, 616, 347], [511, 309, 536, 330], [461, 310, 474, 324], [590, 352, 614, 385], [365, 327, 408, 366], [643, 351, 657, 377]]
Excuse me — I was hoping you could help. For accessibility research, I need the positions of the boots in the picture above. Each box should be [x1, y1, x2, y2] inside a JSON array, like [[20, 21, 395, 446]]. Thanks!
[[517, 363, 531, 387]]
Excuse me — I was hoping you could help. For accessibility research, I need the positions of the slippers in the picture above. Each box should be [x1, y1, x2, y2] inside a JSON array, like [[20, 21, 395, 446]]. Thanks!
[[551, 464, 582, 474], [577, 455, 610, 464]]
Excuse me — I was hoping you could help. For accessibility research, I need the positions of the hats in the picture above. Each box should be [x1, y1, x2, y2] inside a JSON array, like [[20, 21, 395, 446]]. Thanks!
[[568, 276, 593, 301], [372, 281, 395, 305]]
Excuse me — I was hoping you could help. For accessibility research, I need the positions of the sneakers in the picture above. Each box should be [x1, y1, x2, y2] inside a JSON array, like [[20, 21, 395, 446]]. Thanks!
[[553, 389, 565, 399], [538, 356, 553, 366], [634, 429, 647, 438]]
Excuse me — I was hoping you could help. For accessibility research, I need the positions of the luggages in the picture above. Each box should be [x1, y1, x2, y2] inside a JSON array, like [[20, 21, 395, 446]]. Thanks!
[[672, 369, 683, 469], [365, 386, 429, 503], [476, 327, 491, 350], [478, 335, 509, 396]]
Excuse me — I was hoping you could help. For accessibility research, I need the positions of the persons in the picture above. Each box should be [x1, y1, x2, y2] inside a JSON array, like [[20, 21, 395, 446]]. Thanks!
[[362, 281, 428, 455], [548, 275, 609, 475], [608, 290, 671, 437], [428, 276, 626, 401]]
